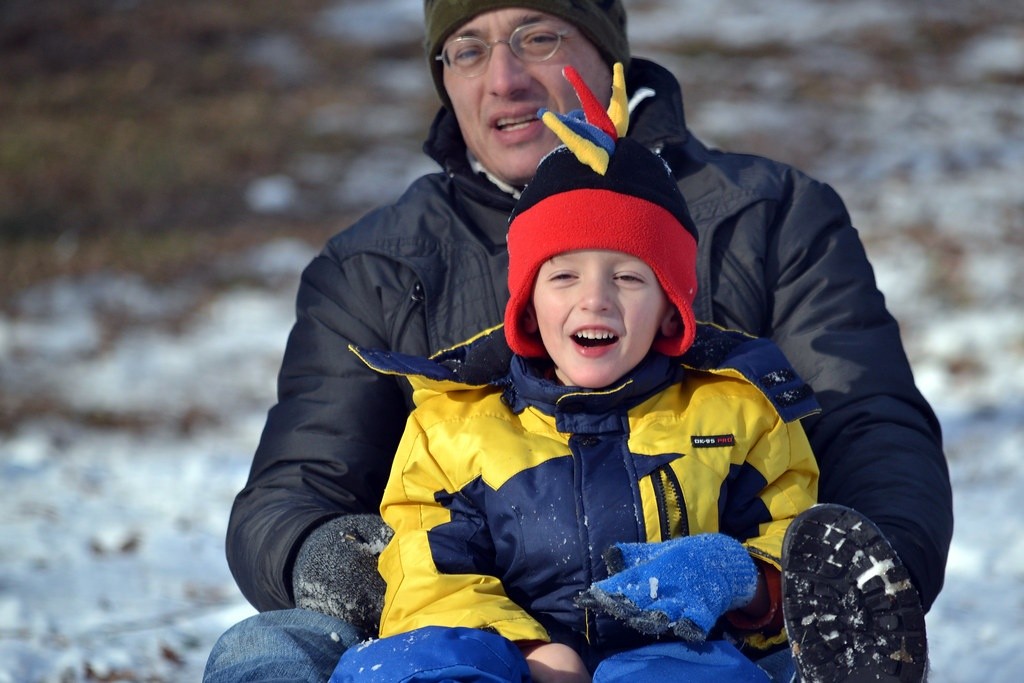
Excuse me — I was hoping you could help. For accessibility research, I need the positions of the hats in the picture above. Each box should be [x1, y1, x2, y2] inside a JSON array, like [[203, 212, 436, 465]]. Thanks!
[[422, 1, 635, 104], [481, 66, 706, 359]]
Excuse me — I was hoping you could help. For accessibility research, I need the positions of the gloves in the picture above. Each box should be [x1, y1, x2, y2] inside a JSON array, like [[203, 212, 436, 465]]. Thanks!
[[592, 530, 765, 646], [288, 512, 396, 630]]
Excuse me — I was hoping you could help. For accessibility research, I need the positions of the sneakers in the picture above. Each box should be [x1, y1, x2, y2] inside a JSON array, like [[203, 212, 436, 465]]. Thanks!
[[781, 505, 932, 683]]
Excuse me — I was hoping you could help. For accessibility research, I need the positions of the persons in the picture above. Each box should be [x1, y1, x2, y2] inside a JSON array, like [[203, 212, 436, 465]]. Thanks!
[[203, 0, 954, 683]]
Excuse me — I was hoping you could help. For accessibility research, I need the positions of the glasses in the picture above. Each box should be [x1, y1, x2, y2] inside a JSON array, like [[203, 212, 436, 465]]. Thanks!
[[427, 15, 580, 83]]
